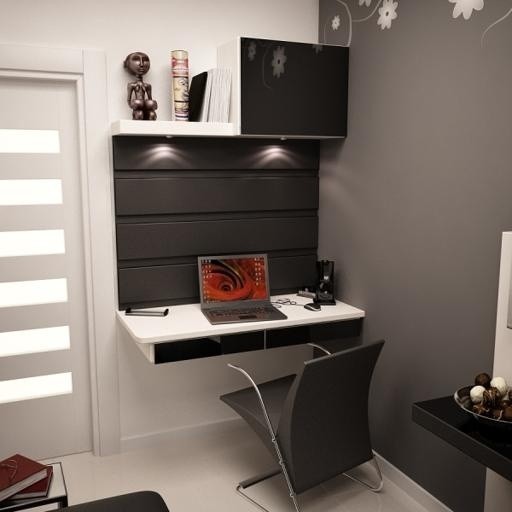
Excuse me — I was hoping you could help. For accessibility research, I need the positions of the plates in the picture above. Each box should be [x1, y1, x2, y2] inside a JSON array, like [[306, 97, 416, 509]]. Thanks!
[[453, 384, 512, 433]]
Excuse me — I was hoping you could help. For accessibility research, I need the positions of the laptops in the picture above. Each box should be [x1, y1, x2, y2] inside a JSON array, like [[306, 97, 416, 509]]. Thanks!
[[197, 253, 287, 326]]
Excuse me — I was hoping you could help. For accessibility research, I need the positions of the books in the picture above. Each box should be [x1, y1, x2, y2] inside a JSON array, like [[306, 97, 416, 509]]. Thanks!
[[1, 453, 49, 503], [10, 463, 54, 500], [188, 66, 233, 122]]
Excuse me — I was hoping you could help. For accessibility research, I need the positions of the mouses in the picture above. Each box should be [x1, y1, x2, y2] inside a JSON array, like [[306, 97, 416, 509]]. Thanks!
[[304, 303, 321, 311]]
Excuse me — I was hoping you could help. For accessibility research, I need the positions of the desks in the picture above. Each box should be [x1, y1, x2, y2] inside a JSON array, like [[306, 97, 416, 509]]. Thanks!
[[114, 293, 367, 368], [411, 396, 512, 484]]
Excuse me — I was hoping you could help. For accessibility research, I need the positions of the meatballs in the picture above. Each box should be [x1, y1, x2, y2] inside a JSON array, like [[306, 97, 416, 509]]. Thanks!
[[475, 373, 491, 390]]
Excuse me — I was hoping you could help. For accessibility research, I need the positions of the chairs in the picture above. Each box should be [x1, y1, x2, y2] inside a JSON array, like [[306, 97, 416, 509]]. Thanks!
[[217, 339, 385, 512]]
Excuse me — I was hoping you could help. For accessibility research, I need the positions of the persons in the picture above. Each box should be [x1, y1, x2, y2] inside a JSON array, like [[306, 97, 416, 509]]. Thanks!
[[125, 52, 158, 120]]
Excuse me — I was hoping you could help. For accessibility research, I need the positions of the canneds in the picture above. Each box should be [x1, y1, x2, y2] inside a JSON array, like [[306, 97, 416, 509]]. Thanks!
[[172, 50, 190, 121]]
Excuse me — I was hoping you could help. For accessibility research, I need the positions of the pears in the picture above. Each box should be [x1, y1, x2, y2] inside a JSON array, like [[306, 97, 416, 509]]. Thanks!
[[470, 386, 486, 403], [490, 377, 506, 396]]
